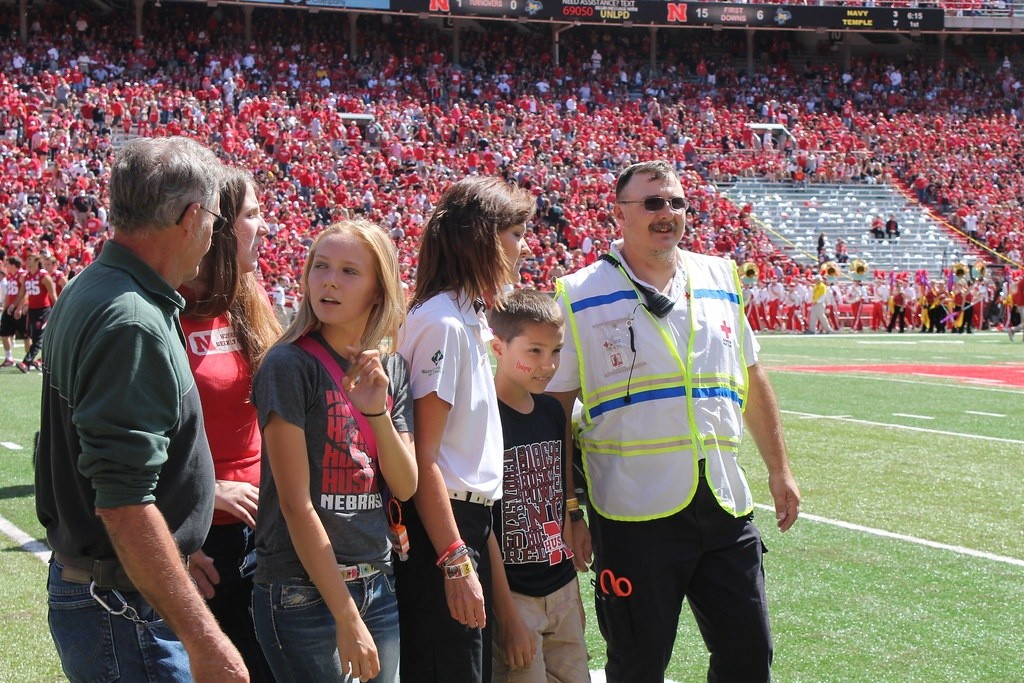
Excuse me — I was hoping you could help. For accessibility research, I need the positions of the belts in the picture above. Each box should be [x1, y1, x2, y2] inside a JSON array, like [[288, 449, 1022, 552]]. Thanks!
[[448, 489, 494, 507], [53, 550, 190, 578], [290, 551, 392, 582]]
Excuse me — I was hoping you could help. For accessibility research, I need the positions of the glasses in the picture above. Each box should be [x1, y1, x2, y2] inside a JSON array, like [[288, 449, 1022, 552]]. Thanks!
[[176, 202, 228, 233], [615, 197, 689, 212]]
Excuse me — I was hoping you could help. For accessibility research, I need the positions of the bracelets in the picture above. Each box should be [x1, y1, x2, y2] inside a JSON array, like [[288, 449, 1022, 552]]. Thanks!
[[566, 498, 584, 522], [437, 539, 474, 580], [362, 401, 389, 417]]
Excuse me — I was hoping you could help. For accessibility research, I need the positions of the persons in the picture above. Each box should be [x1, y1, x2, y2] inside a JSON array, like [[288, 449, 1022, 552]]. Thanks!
[[39, 139, 249, 683], [181, 172, 284, 683], [546, 160, 801, 683], [489, 289, 592, 683], [253, 221, 419, 683], [0, 0, 1024, 374], [391, 175, 538, 683]]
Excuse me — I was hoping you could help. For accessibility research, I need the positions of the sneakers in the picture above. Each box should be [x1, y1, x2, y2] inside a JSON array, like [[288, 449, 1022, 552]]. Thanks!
[[0, 359, 14, 367], [17, 361, 28, 374], [29, 364, 32, 371], [33, 359, 42, 370]]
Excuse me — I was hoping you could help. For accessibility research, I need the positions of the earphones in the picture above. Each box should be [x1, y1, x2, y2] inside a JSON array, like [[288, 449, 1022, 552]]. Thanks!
[[625, 392, 631, 403]]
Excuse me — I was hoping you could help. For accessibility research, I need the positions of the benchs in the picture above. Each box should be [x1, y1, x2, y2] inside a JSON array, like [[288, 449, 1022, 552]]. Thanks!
[[705, 178, 990, 287]]
